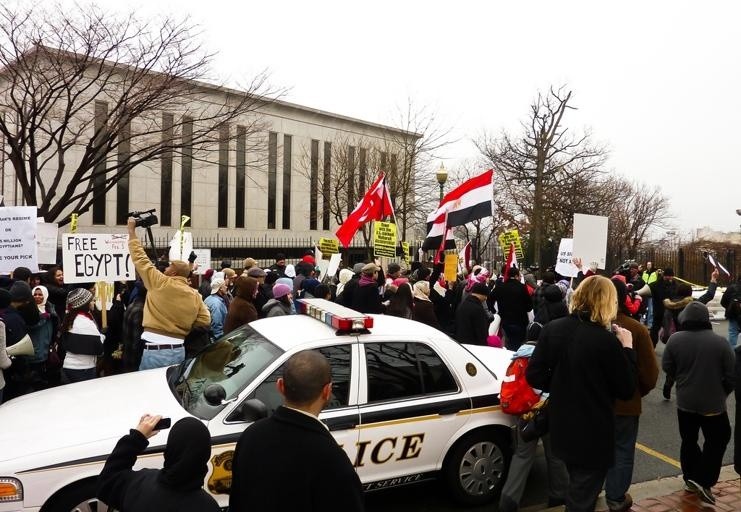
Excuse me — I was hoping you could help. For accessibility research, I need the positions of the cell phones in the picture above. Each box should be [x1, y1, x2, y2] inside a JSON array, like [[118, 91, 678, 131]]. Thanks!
[[152, 418, 171, 430]]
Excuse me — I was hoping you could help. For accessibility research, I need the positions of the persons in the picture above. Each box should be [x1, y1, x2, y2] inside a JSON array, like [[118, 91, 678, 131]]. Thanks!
[[1, 217, 367, 512], [347, 258, 741, 512]]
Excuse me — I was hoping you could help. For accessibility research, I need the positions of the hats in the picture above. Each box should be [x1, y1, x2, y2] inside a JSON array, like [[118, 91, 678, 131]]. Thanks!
[[472, 282, 488, 295], [243, 257, 266, 277], [527, 321, 544, 340], [418, 267, 430, 279], [273, 284, 291, 298], [388, 264, 399, 273], [556, 283, 567, 298], [664, 268, 674, 276], [9, 280, 32, 302], [205, 268, 238, 295], [176, 260, 190, 277], [65, 287, 92, 313], [339, 263, 380, 284], [303, 279, 320, 293]]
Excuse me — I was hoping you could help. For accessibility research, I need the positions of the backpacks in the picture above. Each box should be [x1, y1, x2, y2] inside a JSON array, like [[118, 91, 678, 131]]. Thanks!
[[500, 356, 544, 415]]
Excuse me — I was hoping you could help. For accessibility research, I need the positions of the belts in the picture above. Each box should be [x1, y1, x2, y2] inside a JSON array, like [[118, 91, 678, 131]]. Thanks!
[[145, 343, 184, 350]]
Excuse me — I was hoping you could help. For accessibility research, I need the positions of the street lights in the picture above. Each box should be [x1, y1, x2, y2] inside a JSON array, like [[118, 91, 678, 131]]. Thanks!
[[436, 161, 449, 204]]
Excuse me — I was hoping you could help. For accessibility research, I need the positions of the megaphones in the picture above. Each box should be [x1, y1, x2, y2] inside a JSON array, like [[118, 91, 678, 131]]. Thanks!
[[628, 283, 652, 298]]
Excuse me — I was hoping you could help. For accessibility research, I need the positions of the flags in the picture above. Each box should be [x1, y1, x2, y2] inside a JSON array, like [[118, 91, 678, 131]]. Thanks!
[[336, 171, 393, 249], [427, 167, 494, 235]]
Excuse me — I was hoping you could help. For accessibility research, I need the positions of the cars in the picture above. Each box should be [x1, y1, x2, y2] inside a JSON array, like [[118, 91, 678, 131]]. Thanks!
[[1, 298, 533, 512]]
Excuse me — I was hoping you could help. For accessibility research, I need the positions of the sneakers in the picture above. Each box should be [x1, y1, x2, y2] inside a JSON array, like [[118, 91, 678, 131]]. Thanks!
[[609, 494, 632, 512], [685, 480, 715, 505]]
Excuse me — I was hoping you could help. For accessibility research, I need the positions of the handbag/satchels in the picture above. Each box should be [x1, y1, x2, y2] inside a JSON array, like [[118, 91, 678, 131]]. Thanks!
[[47, 345, 60, 368], [661, 320, 676, 343], [727, 298, 741, 315], [520, 398, 548, 443]]
